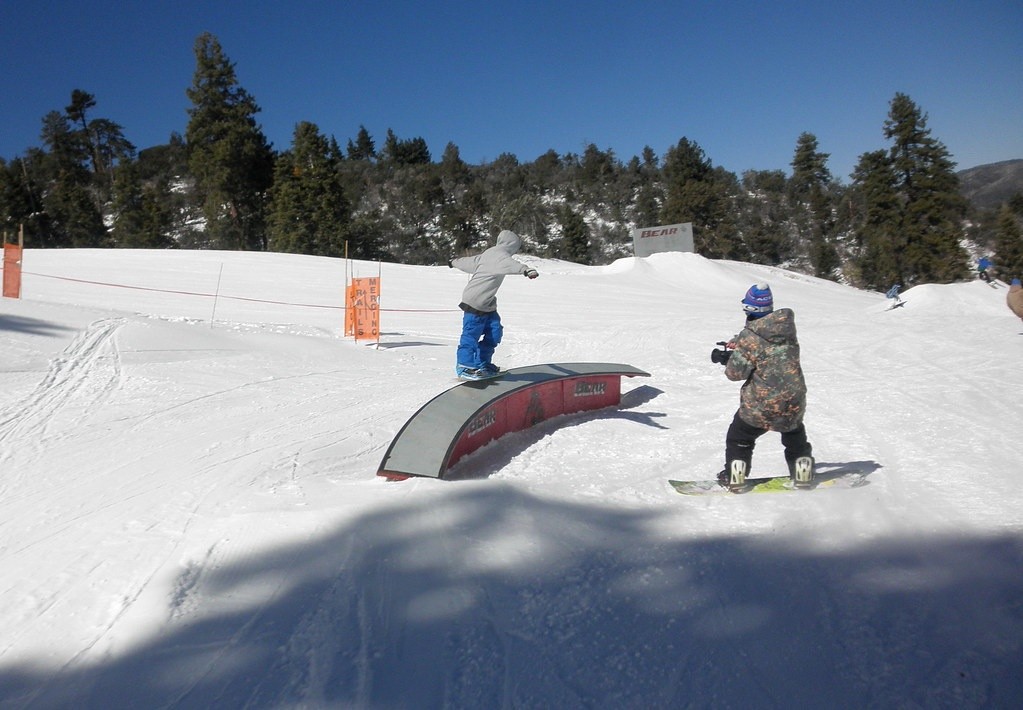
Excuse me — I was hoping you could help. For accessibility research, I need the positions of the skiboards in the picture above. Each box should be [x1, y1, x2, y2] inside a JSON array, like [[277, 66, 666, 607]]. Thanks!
[[887, 301, 907, 310], [986, 280, 1005, 289]]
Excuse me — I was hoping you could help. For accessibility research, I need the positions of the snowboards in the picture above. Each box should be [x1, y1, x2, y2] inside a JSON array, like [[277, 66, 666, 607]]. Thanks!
[[447, 369, 509, 383], [668, 468, 872, 494]]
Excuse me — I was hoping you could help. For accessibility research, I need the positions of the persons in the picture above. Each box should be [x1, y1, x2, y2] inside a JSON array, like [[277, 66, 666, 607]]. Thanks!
[[891, 283, 901, 306], [977, 256, 993, 283], [447, 230, 539, 380], [718, 284, 815, 490]]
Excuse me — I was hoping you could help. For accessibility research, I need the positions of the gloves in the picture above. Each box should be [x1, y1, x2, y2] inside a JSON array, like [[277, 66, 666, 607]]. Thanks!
[[448, 259, 453, 268], [524, 268, 539, 279]]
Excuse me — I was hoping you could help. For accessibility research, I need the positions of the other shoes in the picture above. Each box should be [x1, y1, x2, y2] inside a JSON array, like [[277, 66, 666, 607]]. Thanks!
[[717, 460, 747, 490], [782, 457, 816, 490]]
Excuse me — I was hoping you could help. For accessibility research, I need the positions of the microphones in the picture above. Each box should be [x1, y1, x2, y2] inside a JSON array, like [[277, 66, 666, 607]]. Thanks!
[[717, 341, 727, 346]]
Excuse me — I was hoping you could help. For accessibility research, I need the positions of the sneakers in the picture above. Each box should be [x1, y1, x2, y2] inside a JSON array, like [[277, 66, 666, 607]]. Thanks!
[[474, 363, 500, 377], [459, 368, 487, 381]]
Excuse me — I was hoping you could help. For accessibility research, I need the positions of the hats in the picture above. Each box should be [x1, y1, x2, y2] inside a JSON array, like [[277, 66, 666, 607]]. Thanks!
[[1011, 279, 1020, 286], [740, 282, 773, 317]]
